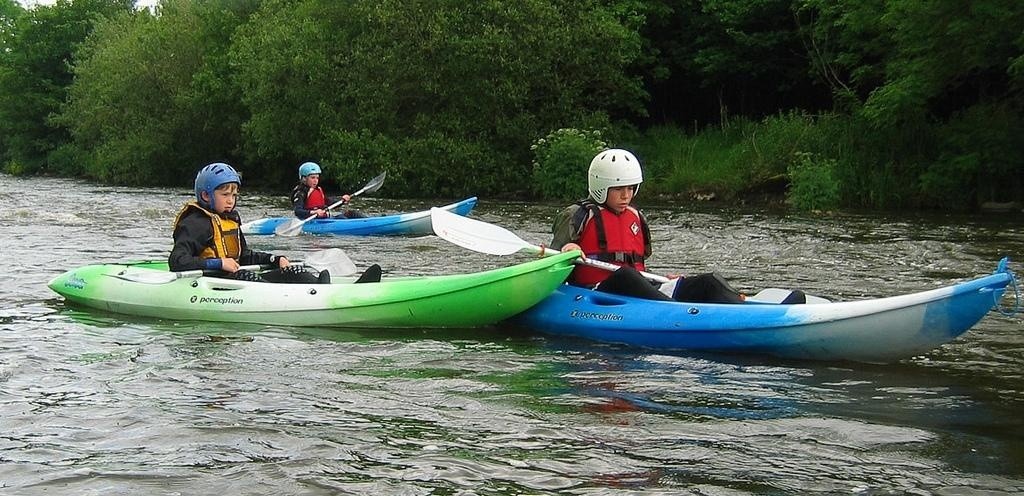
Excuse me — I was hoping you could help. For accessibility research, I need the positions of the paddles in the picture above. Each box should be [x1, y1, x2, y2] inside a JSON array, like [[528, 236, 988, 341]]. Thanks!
[[275, 168, 389, 240], [429, 207, 831, 305], [98, 249, 357, 286]]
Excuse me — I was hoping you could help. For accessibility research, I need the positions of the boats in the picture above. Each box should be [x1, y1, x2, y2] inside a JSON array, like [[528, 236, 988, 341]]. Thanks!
[[48, 248, 577, 326], [234, 197, 479, 236], [527, 258, 1015, 356]]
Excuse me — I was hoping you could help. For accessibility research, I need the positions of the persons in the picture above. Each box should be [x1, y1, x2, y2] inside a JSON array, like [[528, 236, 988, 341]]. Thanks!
[[168, 162, 382, 285], [290, 161, 369, 219], [549, 148, 747, 304]]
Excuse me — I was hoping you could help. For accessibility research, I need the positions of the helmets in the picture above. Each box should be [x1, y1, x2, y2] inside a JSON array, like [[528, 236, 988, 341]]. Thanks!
[[192, 162, 241, 210], [588, 149, 644, 206], [297, 161, 323, 182]]
